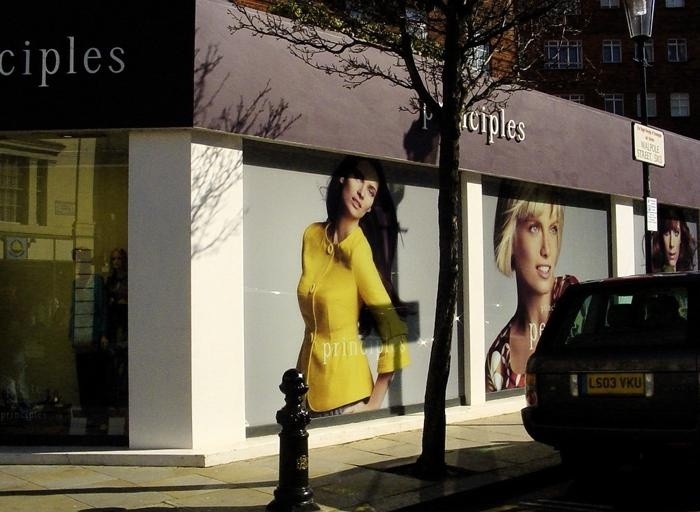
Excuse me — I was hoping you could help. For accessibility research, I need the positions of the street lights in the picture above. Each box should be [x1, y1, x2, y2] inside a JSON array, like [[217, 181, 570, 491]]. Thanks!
[[613, 1, 667, 274]]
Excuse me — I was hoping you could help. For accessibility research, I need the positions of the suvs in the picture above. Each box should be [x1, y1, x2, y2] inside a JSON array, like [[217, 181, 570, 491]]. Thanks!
[[514, 269, 699, 485]]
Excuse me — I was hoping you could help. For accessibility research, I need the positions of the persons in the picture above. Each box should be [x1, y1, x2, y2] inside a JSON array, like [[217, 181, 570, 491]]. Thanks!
[[642, 209, 696, 320], [67, 245, 117, 436], [293, 152, 412, 418], [102, 246, 129, 413], [485, 177, 591, 395]]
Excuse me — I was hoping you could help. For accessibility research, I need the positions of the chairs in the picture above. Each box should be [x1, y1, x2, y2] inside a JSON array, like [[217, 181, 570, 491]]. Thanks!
[[595, 305, 636, 335], [645, 294, 689, 341]]
